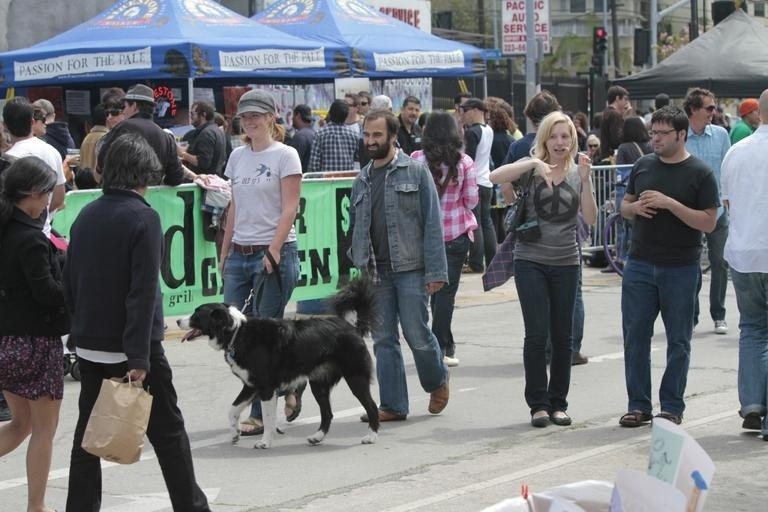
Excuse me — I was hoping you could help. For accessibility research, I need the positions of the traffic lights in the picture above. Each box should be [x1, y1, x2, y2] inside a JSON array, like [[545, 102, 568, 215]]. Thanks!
[[593, 26, 608, 55]]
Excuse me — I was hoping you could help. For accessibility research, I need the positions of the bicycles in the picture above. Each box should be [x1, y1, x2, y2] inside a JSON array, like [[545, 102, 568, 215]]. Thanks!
[[602, 171, 714, 279]]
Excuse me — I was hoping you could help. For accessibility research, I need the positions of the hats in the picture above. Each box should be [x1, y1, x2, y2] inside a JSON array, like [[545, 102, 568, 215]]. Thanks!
[[236, 88, 276, 116], [458, 97, 488, 112], [120, 84, 158, 107], [740, 98, 760, 117], [296, 104, 317, 122]]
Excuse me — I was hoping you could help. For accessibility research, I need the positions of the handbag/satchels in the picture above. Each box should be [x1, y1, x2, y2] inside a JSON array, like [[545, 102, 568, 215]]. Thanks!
[[502, 196, 525, 236], [80, 367, 152, 465]]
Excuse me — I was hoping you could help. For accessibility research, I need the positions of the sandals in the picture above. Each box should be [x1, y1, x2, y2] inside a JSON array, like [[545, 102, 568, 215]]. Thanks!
[[571, 353, 588, 366], [619, 409, 654, 427], [236, 417, 263, 435], [651, 411, 683, 430], [285, 378, 307, 423]]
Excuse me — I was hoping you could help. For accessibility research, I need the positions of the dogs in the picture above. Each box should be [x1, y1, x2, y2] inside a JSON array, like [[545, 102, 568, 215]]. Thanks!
[[176, 270, 383, 450]]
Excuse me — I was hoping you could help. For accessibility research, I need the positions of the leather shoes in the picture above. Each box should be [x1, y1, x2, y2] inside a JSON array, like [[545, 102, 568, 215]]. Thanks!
[[531, 411, 551, 427], [428, 369, 450, 414], [360, 408, 406, 423], [551, 411, 572, 425]]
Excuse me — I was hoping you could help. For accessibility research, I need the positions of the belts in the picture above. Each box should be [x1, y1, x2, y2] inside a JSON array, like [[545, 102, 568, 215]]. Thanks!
[[234, 243, 266, 255]]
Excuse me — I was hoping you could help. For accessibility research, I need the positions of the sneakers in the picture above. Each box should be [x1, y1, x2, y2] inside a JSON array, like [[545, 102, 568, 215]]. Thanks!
[[443, 356, 460, 368], [715, 319, 729, 334], [601, 261, 624, 273], [742, 411, 761, 429]]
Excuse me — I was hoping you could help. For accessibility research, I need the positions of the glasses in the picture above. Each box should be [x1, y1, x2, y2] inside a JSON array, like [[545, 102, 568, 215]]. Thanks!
[[102, 109, 121, 117], [361, 102, 369, 106], [35, 117, 46, 123], [701, 105, 716, 113], [647, 128, 680, 138]]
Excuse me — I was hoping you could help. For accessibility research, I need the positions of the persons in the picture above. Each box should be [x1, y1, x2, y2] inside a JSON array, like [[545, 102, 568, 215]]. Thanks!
[[2, 86, 304, 512], [289, 85, 768, 439]]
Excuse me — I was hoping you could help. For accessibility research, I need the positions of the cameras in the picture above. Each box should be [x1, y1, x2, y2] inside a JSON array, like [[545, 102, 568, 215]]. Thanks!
[[515, 221, 541, 242]]
